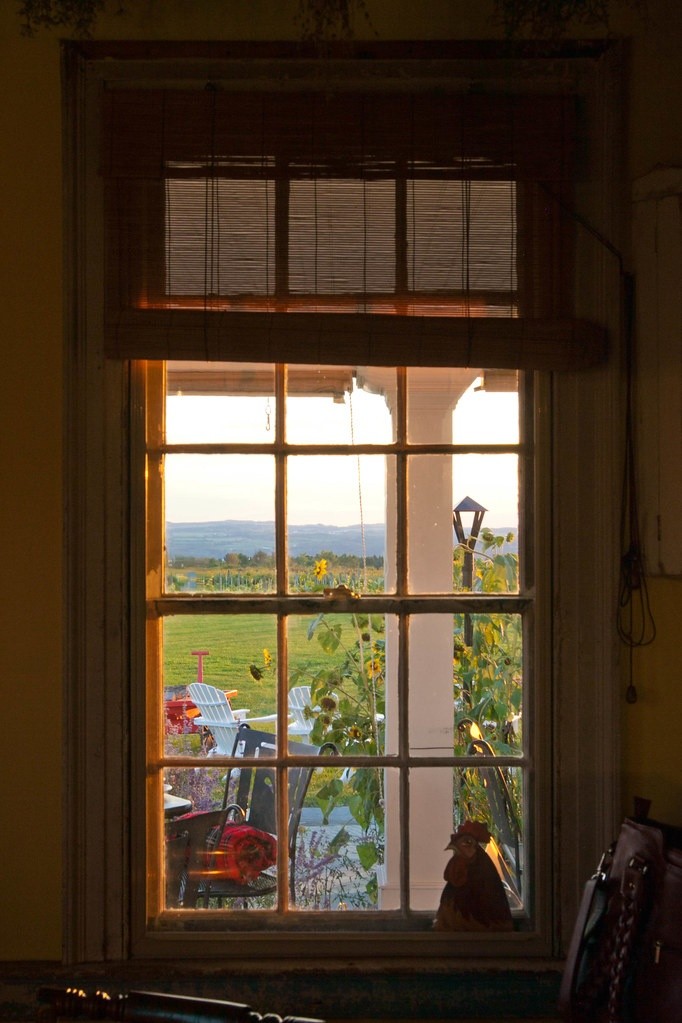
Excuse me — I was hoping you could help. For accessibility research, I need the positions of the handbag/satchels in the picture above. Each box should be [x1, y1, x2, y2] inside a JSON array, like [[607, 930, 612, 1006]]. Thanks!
[[559, 797, 682, 1023]]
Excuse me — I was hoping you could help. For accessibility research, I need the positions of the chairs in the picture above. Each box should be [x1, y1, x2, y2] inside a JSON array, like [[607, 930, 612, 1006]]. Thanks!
[[165, 723, 339, 907], [288, 685, 384, 782], [186, 682, 277, 785], [164, 804, 245, 908]]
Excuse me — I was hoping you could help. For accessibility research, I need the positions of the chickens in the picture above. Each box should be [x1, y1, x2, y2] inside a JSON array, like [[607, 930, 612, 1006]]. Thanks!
[[432, 820, 512, 932]]
